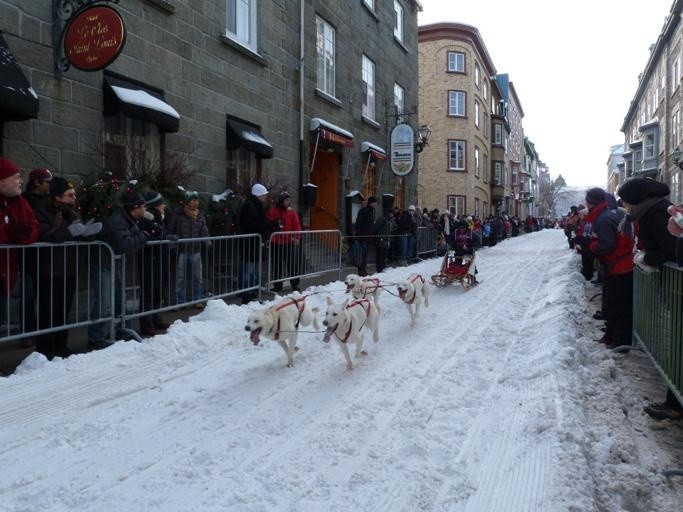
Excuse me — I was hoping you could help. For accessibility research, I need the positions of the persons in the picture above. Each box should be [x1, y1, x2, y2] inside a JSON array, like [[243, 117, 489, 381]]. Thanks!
[[565, 188, 636, 353], [483, 213, 523, 247], [170, 192, 208, 311], [238, 184, 301, 304], [355, 197, 377, 276], [374, 205, 484, 283], [525, 215, 566, 232], [618, 177, 683, 420], [88, 191, 171, 350], [1, 156, 103, 360]]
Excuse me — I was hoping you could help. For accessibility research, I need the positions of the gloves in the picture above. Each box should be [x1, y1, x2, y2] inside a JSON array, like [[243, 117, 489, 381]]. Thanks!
[[142, 224, 161, 240], [68, 218, 102, 236], [6, 223, 32, 241]]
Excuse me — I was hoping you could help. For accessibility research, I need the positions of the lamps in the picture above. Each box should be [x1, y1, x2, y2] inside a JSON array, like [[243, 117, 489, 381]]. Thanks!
[[412, 121, 434, 156], [669, 142, 682, 175]]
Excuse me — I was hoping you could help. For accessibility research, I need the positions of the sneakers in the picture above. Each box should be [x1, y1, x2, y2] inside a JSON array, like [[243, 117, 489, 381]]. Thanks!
[[642, 403, 682, 419]]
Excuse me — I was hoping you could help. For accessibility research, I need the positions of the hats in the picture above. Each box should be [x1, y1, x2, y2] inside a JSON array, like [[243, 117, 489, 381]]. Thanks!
[[617, 178, 669, 204], [29, 168, 73, 194], [278, 191, 290, 202], [184, 188, 198, 204], [251, 183, 268, 197], [123, 190, 162, 209], [0, 157, 19, 180], [585, 188, 617, 209]]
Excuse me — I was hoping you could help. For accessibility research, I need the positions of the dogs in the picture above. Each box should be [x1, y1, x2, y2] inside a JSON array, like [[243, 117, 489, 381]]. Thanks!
[[345, 273, 383, 304], [397, 273, 429, 328], [245, 296, 320, 368], [324, 296, 381, 376]]
